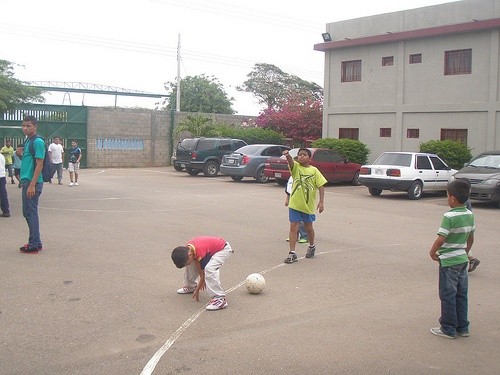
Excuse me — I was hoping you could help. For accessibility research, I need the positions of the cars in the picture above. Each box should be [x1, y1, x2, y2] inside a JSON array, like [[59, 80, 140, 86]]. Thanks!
[[263, 147, 363, 186], [357, 151, 459, 200], [220, 144, 293, 183], [447, 150, 500, 205]]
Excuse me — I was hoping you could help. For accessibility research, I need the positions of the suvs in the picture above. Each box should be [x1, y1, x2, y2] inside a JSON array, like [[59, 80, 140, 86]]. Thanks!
[[173, 136, 251, 177]]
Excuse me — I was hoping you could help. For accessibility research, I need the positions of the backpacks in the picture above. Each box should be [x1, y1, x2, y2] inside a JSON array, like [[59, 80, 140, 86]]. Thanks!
[[29, 133, 53, 182]]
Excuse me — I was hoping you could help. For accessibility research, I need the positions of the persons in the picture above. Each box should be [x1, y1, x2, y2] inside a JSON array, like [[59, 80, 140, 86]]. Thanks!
[[281, 148, 329, 264], [458, 177, 479, 272], [0, 137, 82, 218], [170, 236, 232, 311], [16, 117, 53, 254], [429, 180, 476, 340]]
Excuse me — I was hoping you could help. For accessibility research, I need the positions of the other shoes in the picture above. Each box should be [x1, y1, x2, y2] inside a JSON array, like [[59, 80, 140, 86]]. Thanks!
[[305, 245, 315, 257], [20, 242, 42, 253], [205, 297, 228, 310], [286, 238, 290, 244], [299, 238, 308, 243], [284, 251, 298, 263], [468, 258, 480, 271], [430, 326, 469, 338], [69, 182, 78, 185], [0, 209, 10, 217], [177, 285, 198, 293]]
